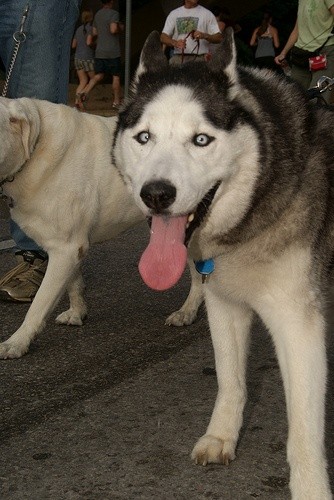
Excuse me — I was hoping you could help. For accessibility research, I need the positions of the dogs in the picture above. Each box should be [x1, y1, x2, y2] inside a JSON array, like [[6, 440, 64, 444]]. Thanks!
[[110, 27, 334, 500], [1, 96, 205, 361]]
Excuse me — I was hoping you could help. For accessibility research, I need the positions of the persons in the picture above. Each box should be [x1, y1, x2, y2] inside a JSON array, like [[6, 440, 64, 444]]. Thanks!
[[1, 1, 79, 304], [249, 12, 279, 72], [77, 0, 126, 108], [160, 0, 224, 68], [272, 0, 334, 108], [71, 9, 98, 109]]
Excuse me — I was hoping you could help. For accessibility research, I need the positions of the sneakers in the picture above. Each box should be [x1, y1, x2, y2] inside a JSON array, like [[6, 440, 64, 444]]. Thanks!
[[0, 249, 50, 302]]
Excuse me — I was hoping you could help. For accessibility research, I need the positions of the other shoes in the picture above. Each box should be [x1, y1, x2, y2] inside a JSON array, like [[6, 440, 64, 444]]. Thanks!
[[112, 102, 122, 109], [77, 92, 87, 112]]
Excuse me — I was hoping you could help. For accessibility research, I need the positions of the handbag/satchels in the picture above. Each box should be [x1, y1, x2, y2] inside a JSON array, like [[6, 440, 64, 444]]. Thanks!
[[308, 46, 334, 71], [287, 47, 320, 70]]
